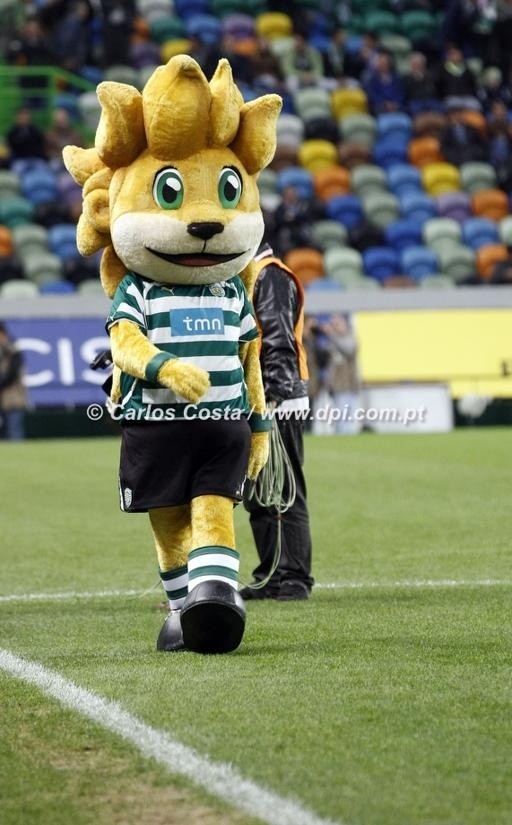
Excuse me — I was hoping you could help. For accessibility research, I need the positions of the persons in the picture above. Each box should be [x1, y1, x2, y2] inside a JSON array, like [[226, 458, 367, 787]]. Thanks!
[[1, 2, 512, 437], [239, 233, 316, 604]]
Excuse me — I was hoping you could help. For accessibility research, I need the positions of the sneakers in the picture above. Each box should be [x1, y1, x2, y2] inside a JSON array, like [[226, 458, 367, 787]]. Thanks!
[[275, 580, 310, 603], [179, 579, 247, 655], [155, 607, 195, 653], [238, 578, 279, 602]]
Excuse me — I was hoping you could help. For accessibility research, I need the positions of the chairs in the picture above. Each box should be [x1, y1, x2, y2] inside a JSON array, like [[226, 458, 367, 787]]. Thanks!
[[0, 0, 512, 298]]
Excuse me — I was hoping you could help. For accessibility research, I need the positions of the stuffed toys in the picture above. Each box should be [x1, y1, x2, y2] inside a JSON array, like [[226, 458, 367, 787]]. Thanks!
[[59, 52, 284, 654]]
